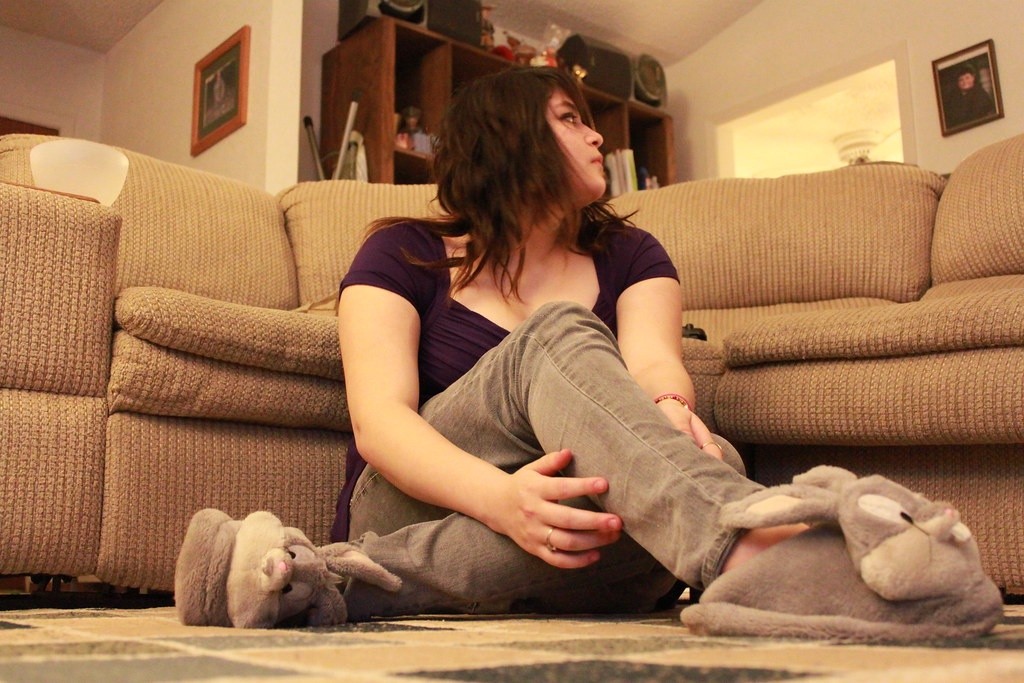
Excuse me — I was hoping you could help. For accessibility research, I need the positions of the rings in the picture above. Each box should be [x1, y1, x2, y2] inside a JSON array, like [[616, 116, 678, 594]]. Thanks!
[[700, 440, 723, 452], [546, 528, 555, 544]]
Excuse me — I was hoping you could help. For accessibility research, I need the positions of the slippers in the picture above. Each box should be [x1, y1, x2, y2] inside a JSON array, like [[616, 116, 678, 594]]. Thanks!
[[175, 508, 400, 629], [679, 465, 1004, 645]]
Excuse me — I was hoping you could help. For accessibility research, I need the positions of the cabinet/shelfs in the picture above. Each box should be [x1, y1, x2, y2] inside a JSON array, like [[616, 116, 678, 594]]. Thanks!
[[321, 20, 675, 208]]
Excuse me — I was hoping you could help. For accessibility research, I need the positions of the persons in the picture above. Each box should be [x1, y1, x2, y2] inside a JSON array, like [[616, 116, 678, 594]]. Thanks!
[[481, 6, 495, 50], [170, 61, 1004, 644], [401, 105, 423, 136]]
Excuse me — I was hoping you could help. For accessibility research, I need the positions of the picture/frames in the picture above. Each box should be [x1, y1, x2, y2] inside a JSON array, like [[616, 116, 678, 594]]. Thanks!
[[931, 39, 1005, 137], [189, 25, 246, 158]]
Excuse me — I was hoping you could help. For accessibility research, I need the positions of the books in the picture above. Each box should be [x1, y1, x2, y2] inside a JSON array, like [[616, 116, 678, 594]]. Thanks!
[[604, 149, 639, 197]]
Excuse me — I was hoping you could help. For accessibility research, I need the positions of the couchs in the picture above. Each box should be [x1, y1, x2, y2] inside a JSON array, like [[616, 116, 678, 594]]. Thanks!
[[1, 135, 1023, 595]]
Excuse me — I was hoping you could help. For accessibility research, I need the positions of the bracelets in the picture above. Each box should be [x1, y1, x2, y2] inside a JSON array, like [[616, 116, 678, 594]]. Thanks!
[[654, 393, 693, 410]]
